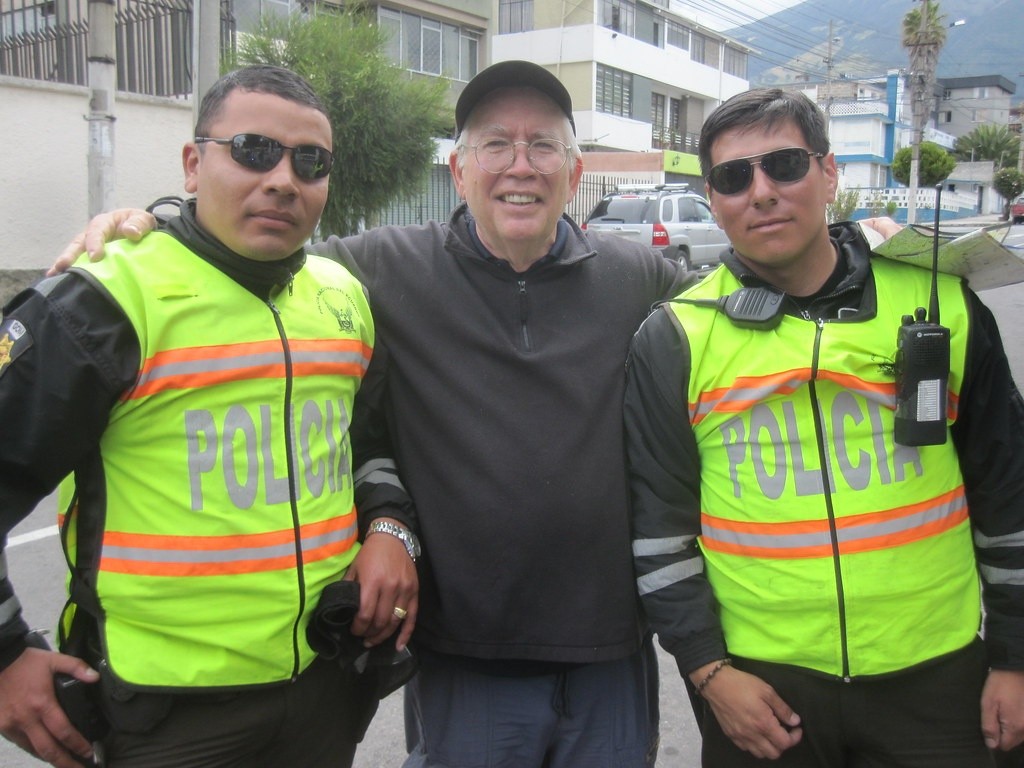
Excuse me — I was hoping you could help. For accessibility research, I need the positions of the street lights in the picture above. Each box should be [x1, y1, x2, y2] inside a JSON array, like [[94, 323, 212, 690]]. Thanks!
[[825, 36, 839, 144], [908, 18, 965, 224]]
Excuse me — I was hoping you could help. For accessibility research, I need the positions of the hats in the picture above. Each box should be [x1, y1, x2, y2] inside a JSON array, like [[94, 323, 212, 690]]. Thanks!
[[453, 60, 575, 143]]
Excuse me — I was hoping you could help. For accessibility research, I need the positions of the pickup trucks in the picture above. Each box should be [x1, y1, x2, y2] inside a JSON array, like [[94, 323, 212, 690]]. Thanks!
[[1011, 197, 1024, 223]]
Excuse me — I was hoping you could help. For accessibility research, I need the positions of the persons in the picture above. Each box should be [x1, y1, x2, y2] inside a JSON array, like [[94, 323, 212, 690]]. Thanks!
[[1, 64, 422, 767], [623, 85, 1023, 768], [49, 61, 903, 768]]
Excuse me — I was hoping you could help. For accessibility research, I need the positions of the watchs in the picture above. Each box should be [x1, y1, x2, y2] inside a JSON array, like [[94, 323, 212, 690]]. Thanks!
[[365, 521, 421, 562]]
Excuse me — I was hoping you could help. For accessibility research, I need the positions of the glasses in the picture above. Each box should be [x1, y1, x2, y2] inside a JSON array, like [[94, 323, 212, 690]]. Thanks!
[[455, 137, 576, 176], [194, 133, 334, 180], [705, 147, 824, 196]]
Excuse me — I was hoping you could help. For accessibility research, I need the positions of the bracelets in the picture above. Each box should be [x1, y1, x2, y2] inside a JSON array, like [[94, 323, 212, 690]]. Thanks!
[[693, 659, 732, 695]]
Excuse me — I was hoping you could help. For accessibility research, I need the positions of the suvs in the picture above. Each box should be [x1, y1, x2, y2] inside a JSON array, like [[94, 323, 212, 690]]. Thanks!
[[584, 186, 731, 272]]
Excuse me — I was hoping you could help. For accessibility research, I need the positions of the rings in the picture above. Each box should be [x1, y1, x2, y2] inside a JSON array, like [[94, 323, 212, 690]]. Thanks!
[[393, 607, 407, 619]]
